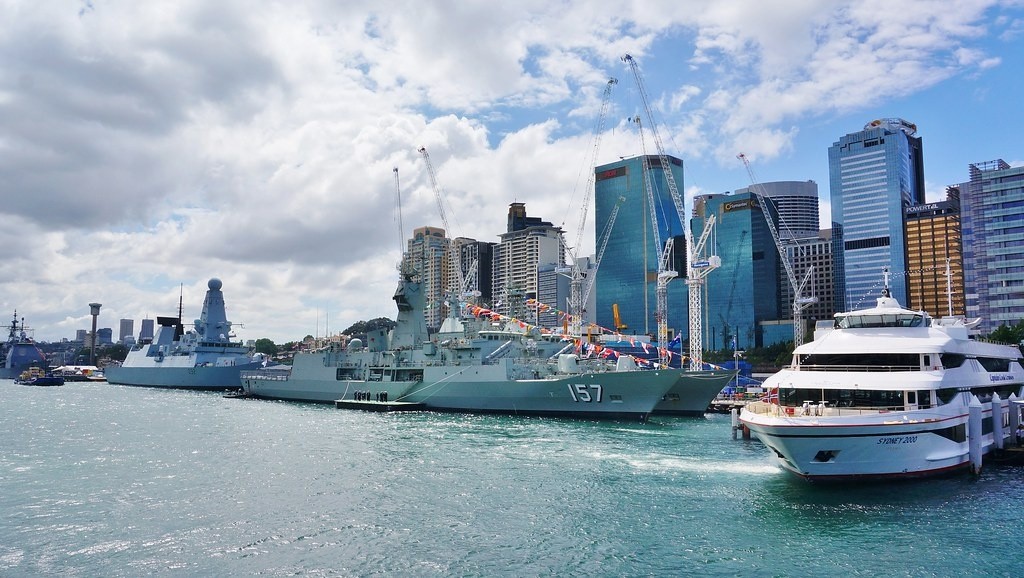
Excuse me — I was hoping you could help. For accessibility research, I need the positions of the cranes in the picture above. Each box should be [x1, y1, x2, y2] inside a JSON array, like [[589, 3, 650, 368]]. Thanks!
[[576, 194, 628, 316], [417, 147, 482, 338], [736, 152, 819, 350], [553, 78, 619, 357], [619, 54, 723, 371]]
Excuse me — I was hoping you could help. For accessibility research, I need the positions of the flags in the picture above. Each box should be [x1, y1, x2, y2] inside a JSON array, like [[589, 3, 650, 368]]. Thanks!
[[730, 335, 737, 352], [759, 386, 779, 405], [261, 356, 268, 367], [667, 330, 682, 351]]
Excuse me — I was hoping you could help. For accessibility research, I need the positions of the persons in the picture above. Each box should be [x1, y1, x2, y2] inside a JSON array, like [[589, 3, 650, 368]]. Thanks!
[[554, 372, 557, 375], [231, 359, 235, 366], [354, 390, 371, 401], [1016, 424, 1024, 448], [869, 393, 930, 410], [376, 390, 387, 402]]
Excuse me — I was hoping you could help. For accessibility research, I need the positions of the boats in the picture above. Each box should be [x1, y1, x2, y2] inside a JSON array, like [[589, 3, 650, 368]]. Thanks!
[[0, 308, 107, 387], [739, 254, 1024, 489], [238, 166, 742, 423], [104, 277, 300, 390]]
